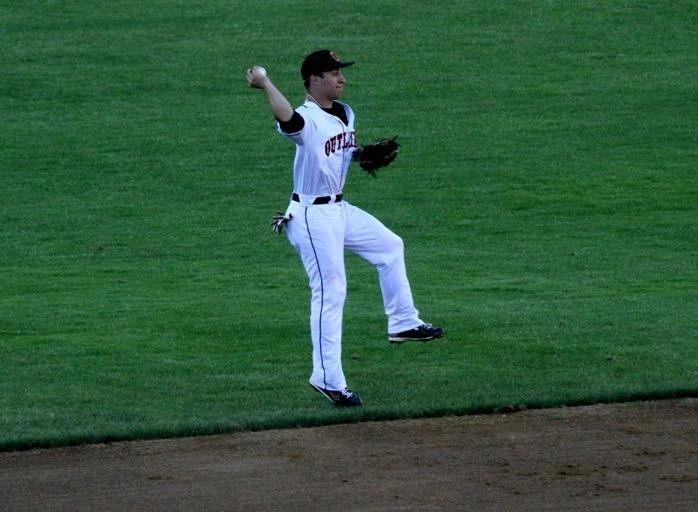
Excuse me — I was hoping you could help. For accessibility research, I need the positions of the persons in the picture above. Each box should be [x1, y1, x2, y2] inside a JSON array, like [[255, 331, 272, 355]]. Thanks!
[[245, 48, 445, 409]]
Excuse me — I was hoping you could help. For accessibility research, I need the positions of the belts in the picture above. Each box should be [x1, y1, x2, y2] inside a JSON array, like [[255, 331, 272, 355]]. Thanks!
[[292, 194, 343, 204]]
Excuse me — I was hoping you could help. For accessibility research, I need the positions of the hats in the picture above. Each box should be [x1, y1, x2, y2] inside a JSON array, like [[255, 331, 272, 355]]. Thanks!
[[301, 50, 355, 79]]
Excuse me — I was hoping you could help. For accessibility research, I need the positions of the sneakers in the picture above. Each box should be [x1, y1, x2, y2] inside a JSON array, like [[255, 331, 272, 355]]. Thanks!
[[388, 324, 445, 342], [308, 379, 363, 408]]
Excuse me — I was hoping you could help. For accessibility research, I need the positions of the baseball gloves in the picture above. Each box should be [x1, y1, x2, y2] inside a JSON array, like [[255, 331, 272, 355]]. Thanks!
[[359, 137, 399, 172]]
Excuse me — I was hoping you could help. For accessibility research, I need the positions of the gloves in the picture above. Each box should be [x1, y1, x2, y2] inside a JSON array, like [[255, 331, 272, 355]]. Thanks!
[[271, 211, 292, 235]]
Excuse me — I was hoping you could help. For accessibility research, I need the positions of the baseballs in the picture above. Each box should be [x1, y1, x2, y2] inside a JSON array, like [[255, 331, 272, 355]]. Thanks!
[[255, 67, 268, 76]]
